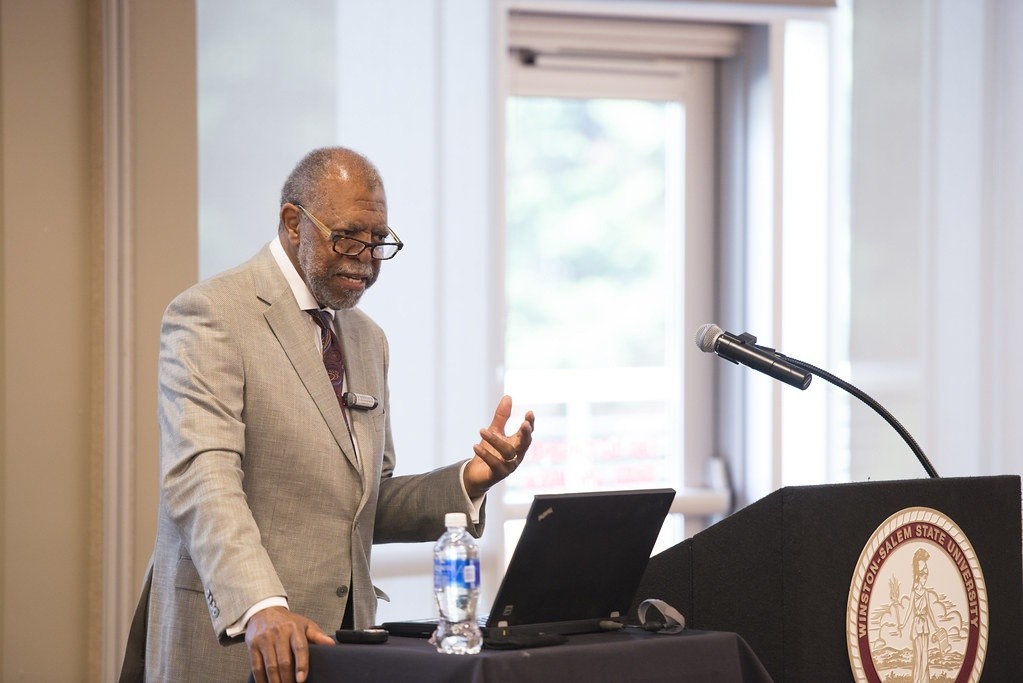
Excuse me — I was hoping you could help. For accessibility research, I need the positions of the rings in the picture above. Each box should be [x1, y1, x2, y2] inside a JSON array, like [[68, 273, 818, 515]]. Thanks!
[[505, 455, 517, 463]]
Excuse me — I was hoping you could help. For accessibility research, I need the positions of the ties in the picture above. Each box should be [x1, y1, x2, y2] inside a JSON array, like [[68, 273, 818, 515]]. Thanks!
[[305, 309, 359, 464]]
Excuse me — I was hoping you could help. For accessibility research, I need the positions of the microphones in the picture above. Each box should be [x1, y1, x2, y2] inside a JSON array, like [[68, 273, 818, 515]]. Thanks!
[[695, 323, 812, 391]]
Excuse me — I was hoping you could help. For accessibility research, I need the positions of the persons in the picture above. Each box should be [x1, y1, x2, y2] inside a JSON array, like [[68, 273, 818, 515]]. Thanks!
[[144, 146, 535, 683]]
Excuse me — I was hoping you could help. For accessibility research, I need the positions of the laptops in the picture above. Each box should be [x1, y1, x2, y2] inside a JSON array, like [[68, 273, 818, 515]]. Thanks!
[[381, 488, 676, 643]]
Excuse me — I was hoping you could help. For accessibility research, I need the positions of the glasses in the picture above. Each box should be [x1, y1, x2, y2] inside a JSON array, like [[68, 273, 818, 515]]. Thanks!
[[293, 203, 404, 260]]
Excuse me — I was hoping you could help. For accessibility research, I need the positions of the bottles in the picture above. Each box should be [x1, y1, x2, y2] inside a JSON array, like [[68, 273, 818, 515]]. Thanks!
[[433, 512, 483, 654]]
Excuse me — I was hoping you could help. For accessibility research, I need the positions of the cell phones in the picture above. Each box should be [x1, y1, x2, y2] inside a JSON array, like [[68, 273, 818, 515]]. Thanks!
[[336, 629, 389, 645]]
